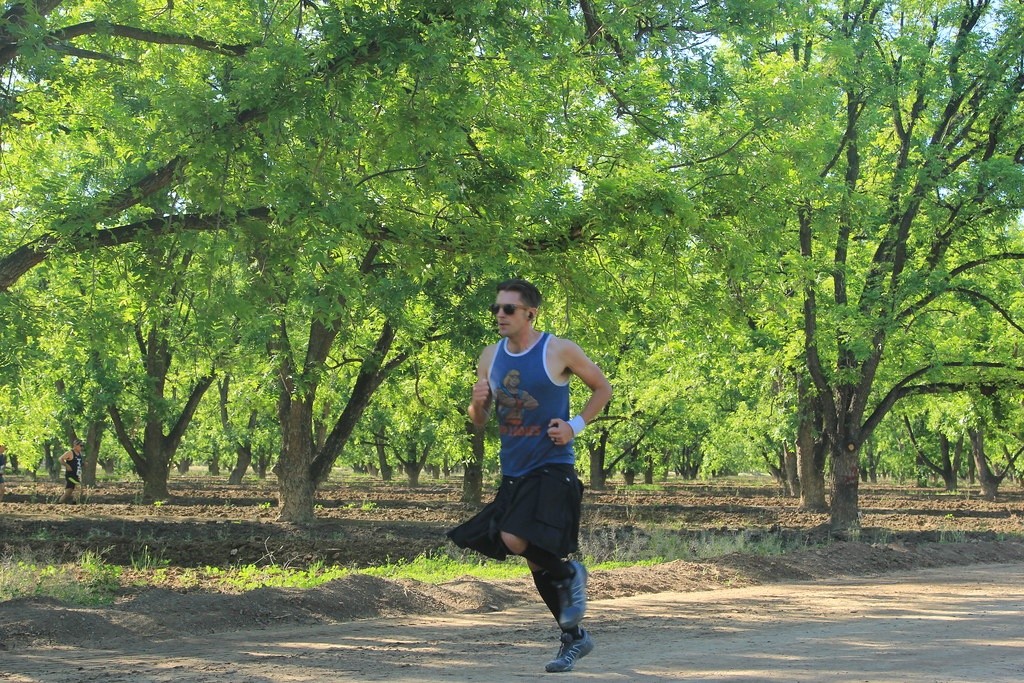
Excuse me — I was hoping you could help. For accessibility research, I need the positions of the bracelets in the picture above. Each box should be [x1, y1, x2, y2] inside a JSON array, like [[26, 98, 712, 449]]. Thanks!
[[567, 414, 585, 438]]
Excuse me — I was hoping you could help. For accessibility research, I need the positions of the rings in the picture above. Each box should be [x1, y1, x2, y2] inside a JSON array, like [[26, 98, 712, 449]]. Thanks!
[[553, 438, 556, 442]]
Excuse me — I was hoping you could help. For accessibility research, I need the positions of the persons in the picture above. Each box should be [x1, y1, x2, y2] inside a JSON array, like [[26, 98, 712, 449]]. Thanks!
[[445, 280, 613, 671], [0, 445, 7, 501], [56, 439, 84, 504]]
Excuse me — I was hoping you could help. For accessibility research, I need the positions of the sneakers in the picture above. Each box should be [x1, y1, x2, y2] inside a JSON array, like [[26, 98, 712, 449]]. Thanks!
[[556, 559, 587, 627], [545, 628, 593, 672]]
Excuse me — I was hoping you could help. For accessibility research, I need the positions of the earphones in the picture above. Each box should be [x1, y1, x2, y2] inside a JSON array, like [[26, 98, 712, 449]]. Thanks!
[[528, 312, 533, 318]]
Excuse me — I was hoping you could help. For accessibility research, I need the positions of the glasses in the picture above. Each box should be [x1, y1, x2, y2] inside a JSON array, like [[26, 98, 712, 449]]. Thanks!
[[77, 444, 84, 448], [489, 303, 528, 315]]
